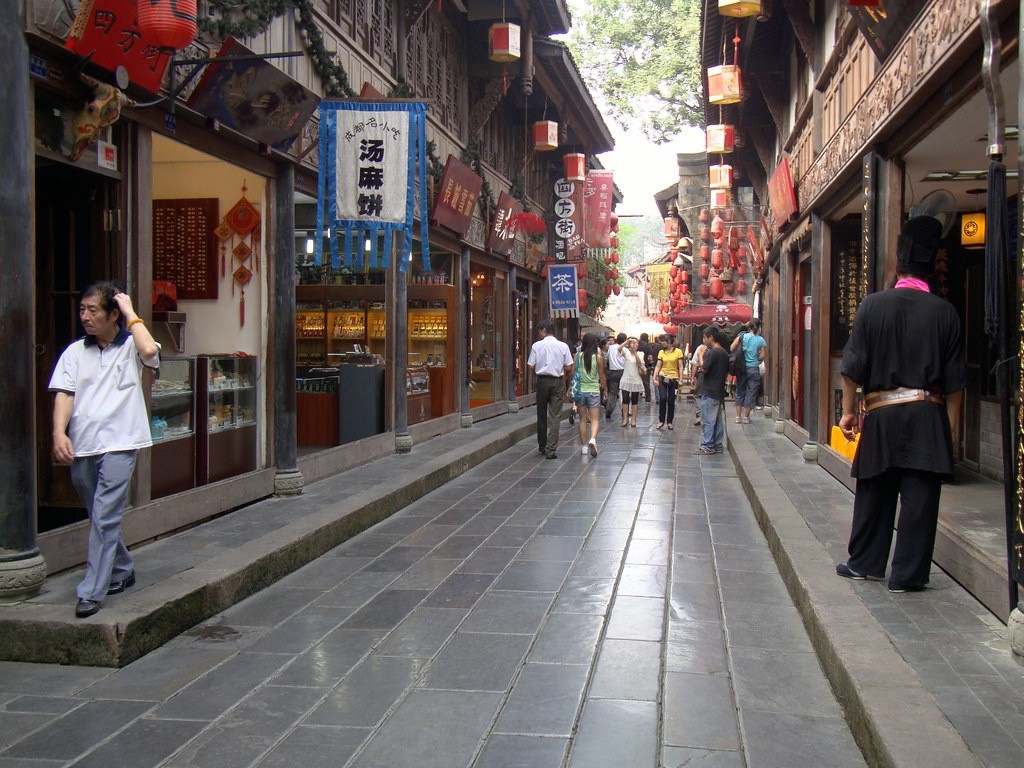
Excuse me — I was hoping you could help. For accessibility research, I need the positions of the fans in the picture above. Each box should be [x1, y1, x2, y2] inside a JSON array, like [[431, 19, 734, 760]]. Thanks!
[[904, 188, 957, 239]]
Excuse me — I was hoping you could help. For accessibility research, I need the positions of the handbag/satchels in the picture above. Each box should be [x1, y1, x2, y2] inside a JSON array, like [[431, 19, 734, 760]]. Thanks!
[[569, 353, 582, 402], [728, 333, 746, 376], [757, 360, 766, 376]]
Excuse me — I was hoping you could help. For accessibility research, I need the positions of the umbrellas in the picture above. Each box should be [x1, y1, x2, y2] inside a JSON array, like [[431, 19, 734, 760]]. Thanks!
[[671, 303, 753, 327]]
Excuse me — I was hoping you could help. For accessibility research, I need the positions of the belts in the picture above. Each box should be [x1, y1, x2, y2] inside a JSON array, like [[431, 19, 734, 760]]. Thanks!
[[864, 387, 945, 415]]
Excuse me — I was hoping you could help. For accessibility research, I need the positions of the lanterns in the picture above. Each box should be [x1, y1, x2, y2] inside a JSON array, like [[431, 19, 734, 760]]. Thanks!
[[563, 152, 586, 183], [706, 125, 734, 154], [489, 22, 522, 96], [709, 165, 733, 190], [576, 211, 620, 314], [961, 213, 986, 250], [717, 0, 763, 71], [657, 190, 747, 334], [138, 0, 196, 55], [707, 65, 743, 106], [533, 121, 558, 151]]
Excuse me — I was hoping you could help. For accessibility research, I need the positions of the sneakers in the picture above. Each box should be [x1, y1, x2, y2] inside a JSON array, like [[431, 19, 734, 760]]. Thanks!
[[589, 439, 598, 456], [581, 444, 589, 454]]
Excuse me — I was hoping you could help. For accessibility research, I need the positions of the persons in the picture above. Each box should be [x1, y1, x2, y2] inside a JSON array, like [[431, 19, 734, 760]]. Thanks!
[[693, 327, 730, 455], [46, 283, 161, 618], [568, 318, 766, 430], [528, 319, 575, 459], [573, 333, 608, 457], [838, 215, 972, 594]]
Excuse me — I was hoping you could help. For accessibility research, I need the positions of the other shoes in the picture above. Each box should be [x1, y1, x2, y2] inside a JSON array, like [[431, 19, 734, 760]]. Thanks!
[[621, 420, 628, 427], [657, 422, 663, 428], [888, 581, 907, 593], [694, 417, 701, 426], [836, 564, 867, 579], [546, 454, 557, 459], [605, 410, 611, 419], [714, 445, 723, 452], [693, 447, 713, 455], [743, 417, 754, 424], [540, 444, 547, 451], [668, 426, 673, 429], [733, 417, 742, 423], [622, 413, 632, 417], [631, 422, 636, 426]]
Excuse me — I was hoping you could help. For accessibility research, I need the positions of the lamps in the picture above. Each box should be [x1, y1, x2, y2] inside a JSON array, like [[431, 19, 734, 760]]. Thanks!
[[960, 188, 990, 251]]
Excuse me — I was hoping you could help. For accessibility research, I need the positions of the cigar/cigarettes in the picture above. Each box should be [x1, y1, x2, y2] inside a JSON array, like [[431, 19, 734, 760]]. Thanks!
[[846, 437, 853, 448]]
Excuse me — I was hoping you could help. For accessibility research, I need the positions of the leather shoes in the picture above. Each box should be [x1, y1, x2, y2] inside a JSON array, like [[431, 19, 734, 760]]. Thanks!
[[75, 598, 100, 615], [108, 570, 136, 593]]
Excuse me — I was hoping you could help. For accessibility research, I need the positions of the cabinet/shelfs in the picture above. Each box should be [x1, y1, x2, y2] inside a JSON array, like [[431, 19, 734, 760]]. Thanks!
[[295, 251, 455, 397], [151, 353, 257, 501]]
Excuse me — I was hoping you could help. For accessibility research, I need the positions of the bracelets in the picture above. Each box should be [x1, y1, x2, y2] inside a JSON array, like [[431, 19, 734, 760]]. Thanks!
[[127, 319, 145, 329]]
